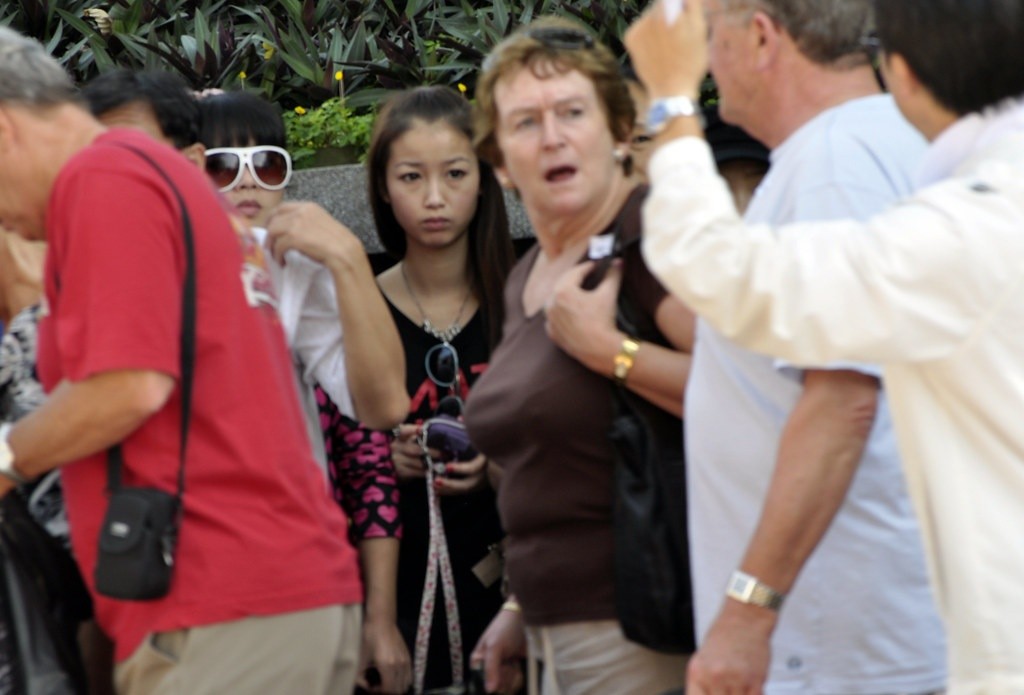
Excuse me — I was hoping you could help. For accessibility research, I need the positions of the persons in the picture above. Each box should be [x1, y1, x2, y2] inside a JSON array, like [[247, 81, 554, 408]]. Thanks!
[[83, 70, 411, 489], [684, 0, 952, 695], [471, 15, 701, 695], [374, 85, 538, 695], [197, 91, 412, 695], [624, 0, 1024, 695], [0, 25, 367, 695]]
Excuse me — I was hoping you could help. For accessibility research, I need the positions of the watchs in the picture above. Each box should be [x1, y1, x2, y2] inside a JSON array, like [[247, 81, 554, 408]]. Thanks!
[[726, 569, 784, 610], [644, 96, 701, 134]]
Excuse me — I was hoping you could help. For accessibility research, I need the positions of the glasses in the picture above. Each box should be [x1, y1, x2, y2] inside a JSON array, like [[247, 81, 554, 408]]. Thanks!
[[425, 339, 465, 422], [203, 145, 292, 194]]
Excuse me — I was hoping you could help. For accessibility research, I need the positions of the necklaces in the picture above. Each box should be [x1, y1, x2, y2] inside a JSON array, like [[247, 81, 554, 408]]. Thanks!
[[400, 258, 472, 342]]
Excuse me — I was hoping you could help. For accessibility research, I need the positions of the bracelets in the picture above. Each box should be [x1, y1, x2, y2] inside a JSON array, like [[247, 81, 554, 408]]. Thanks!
[[613, 336, 639, 378], [1, 422, 26, 484], [503, 602, 519, 611]]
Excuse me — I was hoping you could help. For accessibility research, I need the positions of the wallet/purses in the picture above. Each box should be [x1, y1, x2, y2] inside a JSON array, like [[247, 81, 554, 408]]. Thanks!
[[417, 414, 469, 462]]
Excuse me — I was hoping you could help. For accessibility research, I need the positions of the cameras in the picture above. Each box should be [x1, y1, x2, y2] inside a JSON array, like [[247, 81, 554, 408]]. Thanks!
[[422, 415, 478, 479]]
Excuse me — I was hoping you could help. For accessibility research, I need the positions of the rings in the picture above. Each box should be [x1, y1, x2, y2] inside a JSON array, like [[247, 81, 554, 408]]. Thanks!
[[392, 426, 401, 437]]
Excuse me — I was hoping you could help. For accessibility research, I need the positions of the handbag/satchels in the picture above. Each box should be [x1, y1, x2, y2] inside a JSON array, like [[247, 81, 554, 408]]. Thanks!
[[584, 239, 700, 657]]
